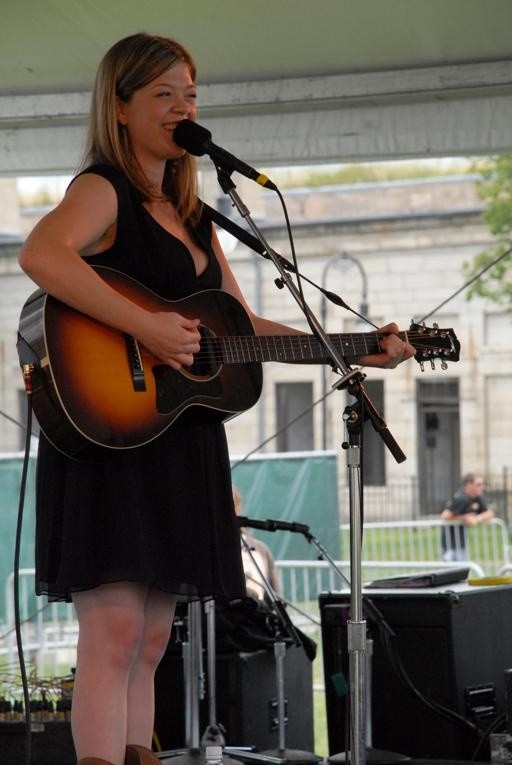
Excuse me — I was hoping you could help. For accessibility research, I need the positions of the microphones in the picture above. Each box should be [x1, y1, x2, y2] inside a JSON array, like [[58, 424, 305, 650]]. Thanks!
[[170, 117, 278, 192]]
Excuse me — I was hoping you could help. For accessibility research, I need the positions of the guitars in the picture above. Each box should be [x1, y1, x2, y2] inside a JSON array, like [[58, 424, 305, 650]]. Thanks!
[[17, 265, 461, 462]]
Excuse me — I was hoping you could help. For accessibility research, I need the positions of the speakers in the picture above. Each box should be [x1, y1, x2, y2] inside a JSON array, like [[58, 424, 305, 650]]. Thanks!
[[318, 585, 512, 764], [158, 641, 316, 754]]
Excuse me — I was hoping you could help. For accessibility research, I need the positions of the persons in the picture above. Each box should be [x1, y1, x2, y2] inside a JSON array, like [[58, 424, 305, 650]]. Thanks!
[[232, 484, 282, 616], [18, 33, 419, 765], [440, 472, 494, 561]]
